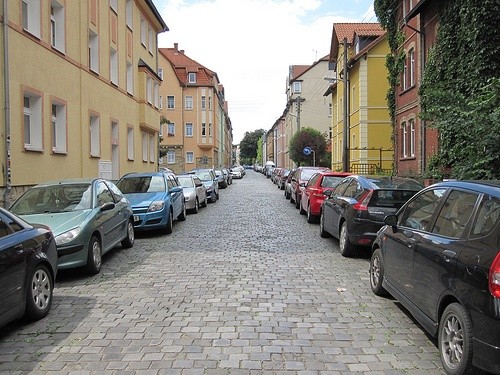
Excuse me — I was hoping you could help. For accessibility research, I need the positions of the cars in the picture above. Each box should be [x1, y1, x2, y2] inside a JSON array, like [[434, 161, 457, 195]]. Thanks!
[[0, 206, 60, 331], [231, 166, 245, 179], [215, 169, 233, 188], [7, 177, 136, 275], [284, 166, 355, 223], [318, 175, 435, 258], [176, 174, 208, 213], [369, 179, 499, 375], [255, 164, 291, 190]]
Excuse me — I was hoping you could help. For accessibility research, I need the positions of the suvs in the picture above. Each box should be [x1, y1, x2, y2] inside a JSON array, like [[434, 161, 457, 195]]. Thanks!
[[116, 171, 186, 234], [188, 168, 219, 203]]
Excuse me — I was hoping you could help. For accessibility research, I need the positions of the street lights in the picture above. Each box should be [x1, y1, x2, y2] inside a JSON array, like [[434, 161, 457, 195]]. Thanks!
[[323, 76, 349, 173]]
